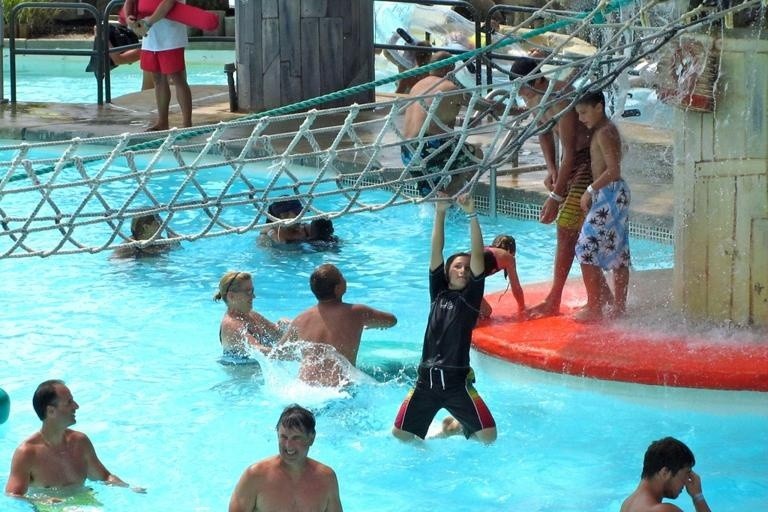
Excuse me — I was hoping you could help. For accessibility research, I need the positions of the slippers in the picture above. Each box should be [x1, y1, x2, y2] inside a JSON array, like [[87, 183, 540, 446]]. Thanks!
[[144, 127, 169, 132]]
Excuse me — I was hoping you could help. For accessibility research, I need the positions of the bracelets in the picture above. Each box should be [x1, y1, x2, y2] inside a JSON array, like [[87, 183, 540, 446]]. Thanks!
[[267, 229, 276, 237], [143, 20, 151, 29], [463, 209, 477, 220], [689, 494, 703, 505], [585, 183, 599, 196], [547, 191, 564, 205]]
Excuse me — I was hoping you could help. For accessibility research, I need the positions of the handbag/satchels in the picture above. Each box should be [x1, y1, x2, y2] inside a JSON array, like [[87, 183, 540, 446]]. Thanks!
[[109, 25, 138, 47]]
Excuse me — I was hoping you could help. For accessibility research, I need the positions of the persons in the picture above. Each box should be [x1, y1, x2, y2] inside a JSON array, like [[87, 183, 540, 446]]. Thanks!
[[258, 193, 311, 252], [391, 189, 497, 446], [91, 25, 187, 90], [401, 49, 531, 210], [381, 32, 419, 74], [213, 271, 297, 365], [109, 209, 183, 260], [259, 217, 338, 248], [271, 263, 397, 388], [568, 84, 635, 325], [464, 233, 525, 321], [228, 403, 345, 512], [507, 59, 615, 319], [122, 0, 194, 131], [394, 40, 432, 95], [619, 435, 713, 512], [4, 379, 150, 511]]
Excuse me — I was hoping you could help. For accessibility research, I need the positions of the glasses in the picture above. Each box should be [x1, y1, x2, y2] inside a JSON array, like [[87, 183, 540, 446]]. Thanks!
[[240, 288, 254, 295]]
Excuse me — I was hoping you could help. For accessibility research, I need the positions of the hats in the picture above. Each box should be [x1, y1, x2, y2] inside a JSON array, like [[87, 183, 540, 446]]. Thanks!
[[271, 196, 310, 217]]
